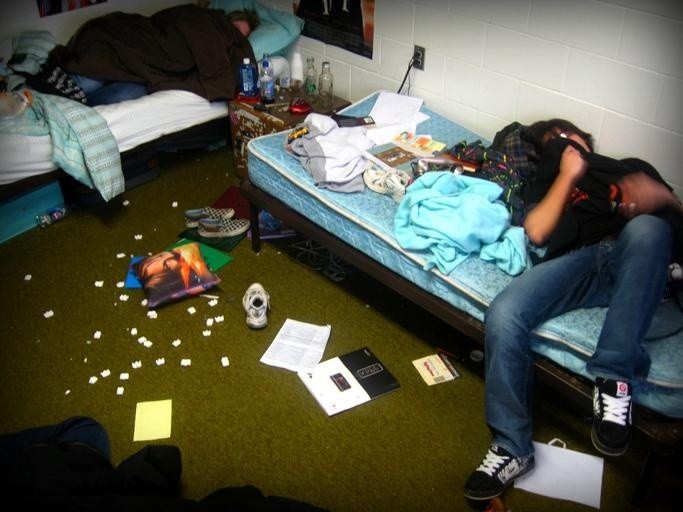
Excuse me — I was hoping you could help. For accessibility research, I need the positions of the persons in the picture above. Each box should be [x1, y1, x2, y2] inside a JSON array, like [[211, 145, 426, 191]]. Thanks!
[[68, 9, 259, 104], [462, 118, 683, 502]]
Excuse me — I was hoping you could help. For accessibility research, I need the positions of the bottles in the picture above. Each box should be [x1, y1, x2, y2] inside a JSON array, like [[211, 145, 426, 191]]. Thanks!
[[35, 204, 76, 228], [240, 58, 258, 96], [279, 45, 304, 90], [259, 53, 275, 103], [304, 56, 318, 103], [318, 62, 334, 107]]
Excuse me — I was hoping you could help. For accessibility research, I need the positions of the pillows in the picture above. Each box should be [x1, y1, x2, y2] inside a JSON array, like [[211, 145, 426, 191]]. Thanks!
[[209, 0, 307, 63], [128, 239, 220, 312]]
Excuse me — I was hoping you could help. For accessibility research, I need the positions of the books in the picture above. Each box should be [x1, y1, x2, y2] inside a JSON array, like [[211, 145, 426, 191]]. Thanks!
[[393, 131, 445, 159], [297, 346, 400, 417], [362, 141, 422, 172]]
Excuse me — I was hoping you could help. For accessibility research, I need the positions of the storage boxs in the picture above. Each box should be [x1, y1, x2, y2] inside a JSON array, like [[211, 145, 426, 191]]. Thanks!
[[0, 175, 66, 245]]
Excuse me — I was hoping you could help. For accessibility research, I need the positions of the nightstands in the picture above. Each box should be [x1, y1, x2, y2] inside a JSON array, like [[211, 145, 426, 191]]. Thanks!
[[226, 80, 352, 179]]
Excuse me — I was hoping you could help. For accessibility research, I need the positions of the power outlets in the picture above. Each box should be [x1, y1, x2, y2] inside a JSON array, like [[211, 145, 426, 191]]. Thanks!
[[412, 46, 425, 70]]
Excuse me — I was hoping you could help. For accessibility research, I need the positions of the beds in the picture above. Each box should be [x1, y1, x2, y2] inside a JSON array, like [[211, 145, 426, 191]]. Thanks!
[[0, 50, 292, 186], [246, 90, 683, 507]]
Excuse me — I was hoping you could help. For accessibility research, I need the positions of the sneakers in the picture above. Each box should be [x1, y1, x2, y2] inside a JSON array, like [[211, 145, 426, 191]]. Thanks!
[[245, 217, 297, 241], [239, 280, 271, 331], [588, 373, 635, 459], [195, 215, 251, 238], [460, 441, 537, 502], [183, 206, 237, 229]]
